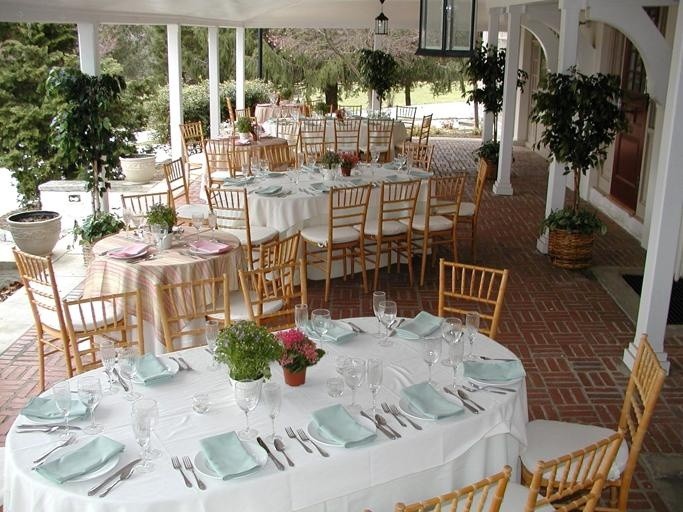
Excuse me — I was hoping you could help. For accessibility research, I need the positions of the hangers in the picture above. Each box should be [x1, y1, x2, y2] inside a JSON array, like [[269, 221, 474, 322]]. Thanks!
[[274, 439, 295, 466], [13, 426, 58, 434], [457, 389, 485, 411], [375, 414, 401, 438]]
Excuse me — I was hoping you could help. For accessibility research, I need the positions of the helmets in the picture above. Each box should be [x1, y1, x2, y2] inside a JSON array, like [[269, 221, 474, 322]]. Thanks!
[[458, 361, 524, 386], [46, 436, 121, 482], [303, 319, 358, 341], [189, 243, 230, 254], [25, 390, 98, 425], [120, 357, 180, 384], [307, 414, 375, 448], [398, 391, 462, 422], [108, 247, 146, 259], [193, 440, 268, 481]]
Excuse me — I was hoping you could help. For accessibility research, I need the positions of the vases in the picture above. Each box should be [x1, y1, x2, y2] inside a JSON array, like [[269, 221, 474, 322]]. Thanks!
[[270, 328, 318, 374], [338, 150, 358, 168]]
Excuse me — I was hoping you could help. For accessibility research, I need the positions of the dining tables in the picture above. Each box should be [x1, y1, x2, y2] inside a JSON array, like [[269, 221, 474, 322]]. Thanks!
[[527, 64, 638, 271], [145, 202, 177, 239], [314, 102, 328, 117], [321, 150, 340, 170], [237, 116, 258, 141], [459, 38, 529, 179], [210, 319, 283, 397], [44, 63, 139, 272]]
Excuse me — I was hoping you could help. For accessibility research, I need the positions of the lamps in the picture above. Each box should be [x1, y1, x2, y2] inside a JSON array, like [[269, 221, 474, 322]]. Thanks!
[[206, 230, 300, 323], [204, 185, 280, 272], [339, 105, 362, 117], [431, 157, 488, 270], [351, 177, 422, 292], [300, 182, 373, 302], [11, 245, 126, 392], [298, 117, 326, 155], [396, 464, 512, 512], [163, 157, 211, 226], [281, 106, 300, 117], [155, 273, 230, 352], [406, 144, 434, 172], [238, 258, 307, 333], [438, 258, 509, 340], [276, 119, 301, 164], [178, 121, 207, 190], [397, 171, 466, 288], [439, 424, 623, 512], [360, 118, 394, 163], [235, 107, 250, 121], [119, 189, 169, 226], [250, 117, 257, 124], [303, 101, 317, 117], [247, 145, 265, 177], [226, 149, 248, 176], [203, 139, 243, 198], [404, 113, 433, 150], [329, 117, 361, 155], [396, 105, 417, 140], [521, 332, 667, 512], [263, 143, 298, 170], [62, 289, 144, 375]]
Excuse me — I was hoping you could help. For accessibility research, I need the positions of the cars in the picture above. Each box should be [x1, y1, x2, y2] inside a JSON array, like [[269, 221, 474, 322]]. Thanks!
[[208, 211, 217, 244], [205, 319, 220, 372], [251, 156, 256, 170], [378, 302, 397, 348], [393, 157, 404, 172], [466, 312, 479, 342], [344, 358, 363, 412], [447, 337, 471, 392], [116, 348, 142, 401], [100, 341, 119, 394], [307, 155, 316, 168], [325, 378, 344, 399], [242, 163, 248, 181], [122, 208, 131, 235], [366, 360, 382, 414], [54, 383, 75, 439], [440, 316, 460, 368], [132, 399, 156, 472], [133, 214, 146, 229], [192, 212, 204, 243], [371, 150, 380, 166], [422, 335, 441, 387], [263, 384, 282, 444], [78, 379, 102, 435], [372, 291, 385, 336], [295, 304, 307, 329], [153, 223, 162, 245], [312, 308, 330, 353], [235, 379, 260, 440], [191, 394, 211, 414], [260, 160, 269, 174]]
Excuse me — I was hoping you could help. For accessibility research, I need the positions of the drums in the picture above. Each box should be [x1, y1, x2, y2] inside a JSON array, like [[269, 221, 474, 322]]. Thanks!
[[87, 458, 142, 496], [389, 317, 406, 339], [15, 424, 82, 431], [443, 387, 480, 416], [256, 437, 286, 471], [360, 411, 396, 440]]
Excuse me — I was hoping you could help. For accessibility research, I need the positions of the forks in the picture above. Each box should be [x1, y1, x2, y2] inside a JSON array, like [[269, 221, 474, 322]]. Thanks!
[[170, 456, 193, 488], [389, 404, 422, 430], [285, 427, 314, 453], [182, 456, 205, 490], [298, 430, 328, 457], [99, 468, 133, 497], [33, 435, 75, 464], [381, 402, 406, 427]]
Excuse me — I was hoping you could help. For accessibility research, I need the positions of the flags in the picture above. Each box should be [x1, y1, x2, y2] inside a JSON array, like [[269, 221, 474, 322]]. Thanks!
[[340, 167, 352, 176], [282, 366, 306, 387]]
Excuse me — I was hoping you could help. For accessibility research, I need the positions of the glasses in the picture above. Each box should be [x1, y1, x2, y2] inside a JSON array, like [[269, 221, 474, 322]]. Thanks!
[[415, 0, 477, 57], [374, 0, 390, 36]]
